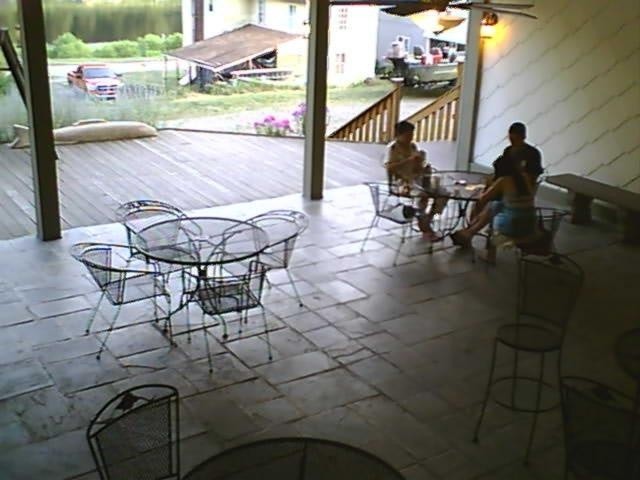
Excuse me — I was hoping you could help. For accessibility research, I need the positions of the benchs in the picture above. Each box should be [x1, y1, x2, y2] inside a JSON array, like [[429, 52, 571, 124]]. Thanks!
[[548, 174, 639, 242]]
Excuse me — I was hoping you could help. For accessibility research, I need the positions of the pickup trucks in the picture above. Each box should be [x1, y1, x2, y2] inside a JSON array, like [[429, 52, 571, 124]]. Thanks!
[[64, 59, 129, 102]]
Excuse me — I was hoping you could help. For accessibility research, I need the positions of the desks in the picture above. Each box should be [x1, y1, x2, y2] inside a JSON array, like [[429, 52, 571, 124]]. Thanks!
[[614, 325, 638, 376], [136, 216, 270, 334], [181, 436, 406, 480]]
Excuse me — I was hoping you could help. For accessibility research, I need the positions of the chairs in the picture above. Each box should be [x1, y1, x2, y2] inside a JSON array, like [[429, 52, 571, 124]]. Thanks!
[[189, 261, 274, 373], [547, 376, 639, 478], [112, 200, 200, 296], [472, 253, 584, 463], [216, 208, 305, 306], [83, 385, 181, 479], [358, 136, 571, 265], [70, 243, 172, 358]]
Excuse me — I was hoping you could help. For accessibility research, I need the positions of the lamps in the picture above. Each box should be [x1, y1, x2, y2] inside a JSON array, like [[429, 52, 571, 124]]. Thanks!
[[438, 5, 467, 29]]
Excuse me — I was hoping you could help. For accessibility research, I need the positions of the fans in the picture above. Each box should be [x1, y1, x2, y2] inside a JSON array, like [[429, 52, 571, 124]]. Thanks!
[[327, 0, 539, 24]]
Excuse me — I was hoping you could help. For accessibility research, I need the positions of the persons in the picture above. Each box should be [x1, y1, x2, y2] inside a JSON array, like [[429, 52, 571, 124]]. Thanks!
[[382, 119, 451, 243], [449, 146, 537, 264], [467, 120, 544, 229]]
